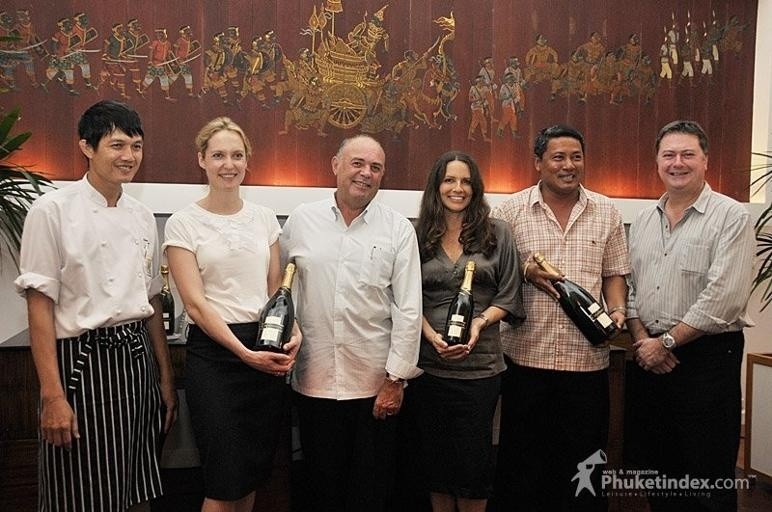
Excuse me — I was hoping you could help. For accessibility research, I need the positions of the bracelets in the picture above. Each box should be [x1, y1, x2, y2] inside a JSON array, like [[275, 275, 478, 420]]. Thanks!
[[607, 305, 628, 316], [473, 313, 489, 331]]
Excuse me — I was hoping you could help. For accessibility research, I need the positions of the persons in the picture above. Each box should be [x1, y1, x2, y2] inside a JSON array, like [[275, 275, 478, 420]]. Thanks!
[[489, 123, 632, 511], [624, 120, 756, 512], [386, 150, 526, 511], [159, 117, 302, 512], [11, 97, 180, 512], [274, 136, 425, 512]]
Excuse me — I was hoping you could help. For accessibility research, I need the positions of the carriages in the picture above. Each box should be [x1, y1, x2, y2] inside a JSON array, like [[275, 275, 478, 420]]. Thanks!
[[300, 0, 456, 136]]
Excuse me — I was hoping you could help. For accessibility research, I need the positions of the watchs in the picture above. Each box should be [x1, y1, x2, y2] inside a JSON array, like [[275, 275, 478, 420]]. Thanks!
[[661, 331, 676, 351], [384, 372, 405, 385]]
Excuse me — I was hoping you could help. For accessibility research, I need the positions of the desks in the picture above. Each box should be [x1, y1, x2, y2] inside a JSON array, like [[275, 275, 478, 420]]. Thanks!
[[0, 324, 630, 510]]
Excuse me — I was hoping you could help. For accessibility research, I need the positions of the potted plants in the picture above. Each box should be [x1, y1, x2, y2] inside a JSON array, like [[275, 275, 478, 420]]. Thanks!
[[740, 138, 771, 488]]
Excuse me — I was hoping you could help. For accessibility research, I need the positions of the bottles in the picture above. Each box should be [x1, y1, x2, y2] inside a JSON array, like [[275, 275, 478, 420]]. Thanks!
[[255, 263, 301, 354], [445, 261, 478, 345], [531, 251, 618, 346], [160, 264, 175, 337]]
